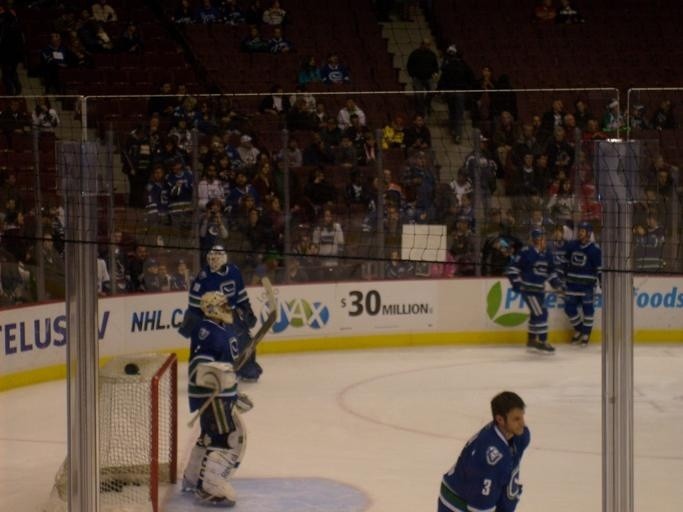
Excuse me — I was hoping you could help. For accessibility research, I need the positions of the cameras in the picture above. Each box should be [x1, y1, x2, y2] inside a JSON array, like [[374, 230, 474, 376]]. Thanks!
[[208, 207, 218, 222]]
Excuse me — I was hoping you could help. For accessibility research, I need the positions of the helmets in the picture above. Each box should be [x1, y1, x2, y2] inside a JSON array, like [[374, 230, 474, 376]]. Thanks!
[[206, 246, 228, 273], [577, 222, 593, 232], [198, 290, 235, 326], [529, 230, 547, 239]]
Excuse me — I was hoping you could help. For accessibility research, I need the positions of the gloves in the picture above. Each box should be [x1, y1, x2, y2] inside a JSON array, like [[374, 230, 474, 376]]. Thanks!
[[554, 285, 567, 297], [241, 311, 257, 329], [512, 282, 528, 293], [179, 319, 197, 338]]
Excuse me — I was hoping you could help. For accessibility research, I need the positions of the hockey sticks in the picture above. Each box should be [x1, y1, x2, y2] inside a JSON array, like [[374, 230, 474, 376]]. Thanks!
[[188, 276, 277, 428]]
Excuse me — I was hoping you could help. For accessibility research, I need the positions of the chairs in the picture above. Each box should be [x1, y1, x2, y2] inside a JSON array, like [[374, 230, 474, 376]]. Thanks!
[[0, 1, 683, 214]]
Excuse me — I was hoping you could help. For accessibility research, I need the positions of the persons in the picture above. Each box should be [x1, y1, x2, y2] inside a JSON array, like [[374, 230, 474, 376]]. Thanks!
[[181, 291, 254, 503], [436, 391, 530, 512], [177, 245, 262, 379], [506, 229, 563, 351], [555, 221, 603, 345], [0, 0, 682, 306]]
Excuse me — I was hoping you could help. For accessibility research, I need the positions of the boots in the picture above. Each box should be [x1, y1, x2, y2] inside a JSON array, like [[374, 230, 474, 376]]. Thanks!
[[536, 342, 554, 352], [527, 341, 540, 348], [571, 331, 581, 341], [581, 333, 589, 344]]
